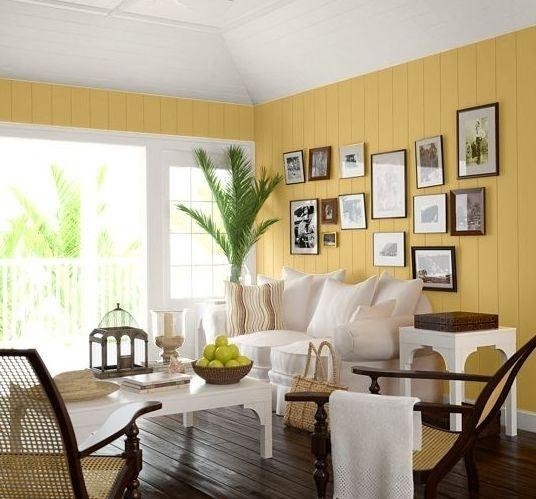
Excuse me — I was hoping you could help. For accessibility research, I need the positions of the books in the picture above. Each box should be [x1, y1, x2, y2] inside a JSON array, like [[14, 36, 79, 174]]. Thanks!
[[121, 370, 192, 392]]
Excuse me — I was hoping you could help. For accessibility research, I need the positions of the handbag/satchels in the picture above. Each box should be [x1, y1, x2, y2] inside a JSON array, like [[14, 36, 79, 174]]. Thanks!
[[283, 342, 348, 436]]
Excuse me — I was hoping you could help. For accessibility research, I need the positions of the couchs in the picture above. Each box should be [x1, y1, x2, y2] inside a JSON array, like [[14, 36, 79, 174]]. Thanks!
[[201, 291, 444, 406]]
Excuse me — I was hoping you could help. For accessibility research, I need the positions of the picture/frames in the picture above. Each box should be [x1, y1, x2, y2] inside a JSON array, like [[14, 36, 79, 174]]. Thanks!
[[371, 231, 407, 268], [454, 102, 501, 181], [448, 186, 488, 237], [288, 197, 320, 256], [337, 141, 367, 180], [320, 197, 339, 226], [337, 192, 368, 231], [321, 231, 339, 249], [370, 148, 408, 220], [413, 134, 446, 190], [282, 149, 307, 186], [306, 144, 331, 181], [412, 192, 448, 235], [409, 245, 459, 293]]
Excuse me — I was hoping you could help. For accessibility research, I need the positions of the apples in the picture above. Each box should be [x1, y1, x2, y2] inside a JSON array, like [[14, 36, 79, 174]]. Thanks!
[[215, 345, 233, 363], [225, 359, 242, 367], [196, 357, 209, 367], [203, 344, 218, 361], [229, 344, 239, 359], [215, 335, 228, 347], [236, 356, 251, 366], [208, 359, 224, 367]]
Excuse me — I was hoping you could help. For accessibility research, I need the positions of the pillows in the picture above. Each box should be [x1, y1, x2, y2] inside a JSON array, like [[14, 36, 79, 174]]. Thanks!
[[223, 278, 288, 337], [348, 298, 398, 322], [282, 267, 347, 332], [305, 275, 380, 339], [370, 268, 424, 318]]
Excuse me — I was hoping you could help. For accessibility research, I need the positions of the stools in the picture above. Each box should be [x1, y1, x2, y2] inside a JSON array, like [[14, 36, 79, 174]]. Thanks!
[[397, 310, 519, 438]]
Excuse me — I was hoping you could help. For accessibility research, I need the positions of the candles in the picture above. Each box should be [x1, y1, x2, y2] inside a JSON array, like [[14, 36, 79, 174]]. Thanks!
[[164, 311, 173, 338]]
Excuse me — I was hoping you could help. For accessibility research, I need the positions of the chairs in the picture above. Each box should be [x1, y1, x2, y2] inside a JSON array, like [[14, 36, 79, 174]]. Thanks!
[[283, 334, 536, 499], [1, 347, 164, 499]]
